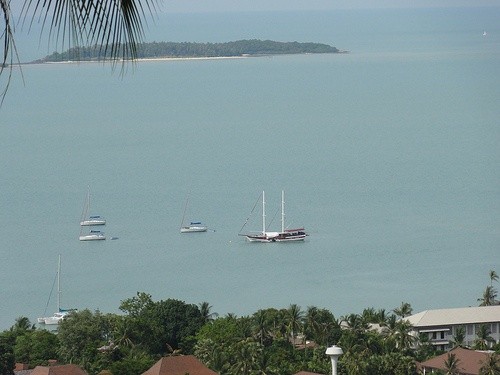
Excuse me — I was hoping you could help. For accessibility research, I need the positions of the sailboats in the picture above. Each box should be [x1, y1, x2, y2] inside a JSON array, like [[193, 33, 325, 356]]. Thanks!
[[237, 188, 310, 243], [79, 184, 106, 226], [180, 198, 208, 234], [36, 254, 79, 325]]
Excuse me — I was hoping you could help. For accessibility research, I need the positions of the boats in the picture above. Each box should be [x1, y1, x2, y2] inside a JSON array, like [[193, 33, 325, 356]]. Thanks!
[[79, 228, 107, 241]]
